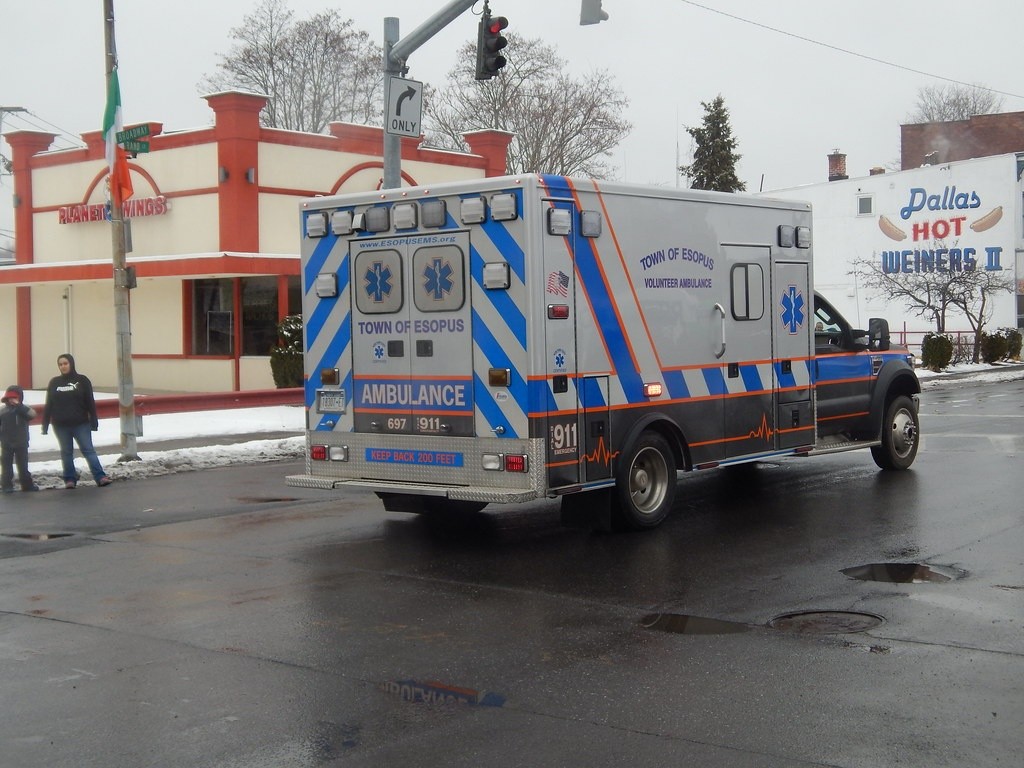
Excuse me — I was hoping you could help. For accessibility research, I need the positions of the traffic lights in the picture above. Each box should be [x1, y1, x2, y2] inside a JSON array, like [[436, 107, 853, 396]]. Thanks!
[[475, 17, 509, 82]]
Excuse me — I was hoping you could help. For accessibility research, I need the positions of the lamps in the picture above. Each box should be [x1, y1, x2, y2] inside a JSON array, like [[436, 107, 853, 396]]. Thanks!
[[219, 165, 229, 183], [245, 168, 255, 184], [12, 193, 21, 208]]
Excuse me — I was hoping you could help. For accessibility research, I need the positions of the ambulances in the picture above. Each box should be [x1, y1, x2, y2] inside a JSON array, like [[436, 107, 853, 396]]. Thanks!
[[286, 173, 923, 530]]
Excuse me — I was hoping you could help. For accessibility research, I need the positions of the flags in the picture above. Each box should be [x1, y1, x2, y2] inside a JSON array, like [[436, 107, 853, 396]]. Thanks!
[[102, 61, 134, 215]]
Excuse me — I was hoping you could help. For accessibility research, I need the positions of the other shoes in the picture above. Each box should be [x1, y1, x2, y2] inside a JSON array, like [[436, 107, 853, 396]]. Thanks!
[[96, 476, 113, 487], [21, 482, 39, 491], [2, 482, 13, 493], [66, 480, 75, 489]]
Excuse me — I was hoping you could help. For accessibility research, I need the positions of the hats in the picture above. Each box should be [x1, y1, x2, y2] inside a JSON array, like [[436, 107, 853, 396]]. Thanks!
[[4, 390, 20, 399]]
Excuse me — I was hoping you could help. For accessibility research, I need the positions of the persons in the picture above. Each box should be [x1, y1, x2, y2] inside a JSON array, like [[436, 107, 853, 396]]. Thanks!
[[42, 354, 114, 490], [0, 385, 39, 492]]
